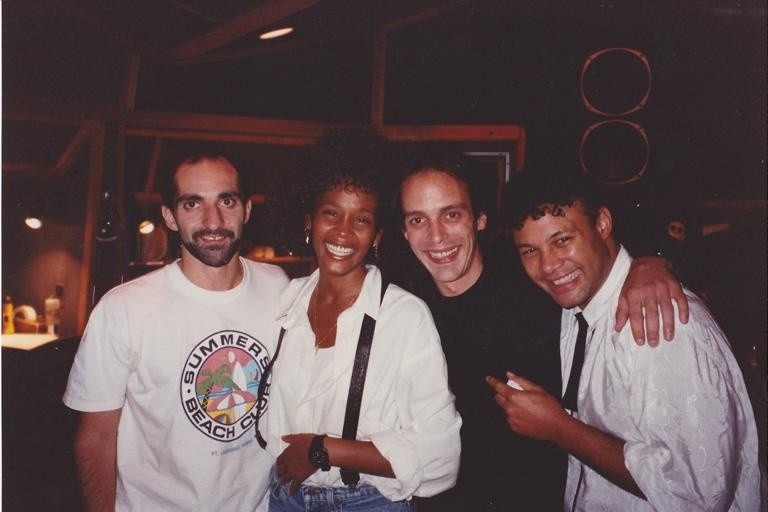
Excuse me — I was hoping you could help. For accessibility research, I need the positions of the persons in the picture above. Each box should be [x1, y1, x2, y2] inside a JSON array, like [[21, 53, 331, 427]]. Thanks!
[[268, 127, 463, 512], [485, 162, 767, 511], [390, 157, 689, 511], [61, 148, 292, 512]]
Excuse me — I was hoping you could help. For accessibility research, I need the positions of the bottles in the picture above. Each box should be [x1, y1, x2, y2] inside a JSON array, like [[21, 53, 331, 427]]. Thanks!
[[44, 292, 60, 333], [2, 296, 15, 335]]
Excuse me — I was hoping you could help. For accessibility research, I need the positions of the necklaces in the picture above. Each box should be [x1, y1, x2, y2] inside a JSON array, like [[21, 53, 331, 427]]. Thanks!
[[314, 283, 358, 354]]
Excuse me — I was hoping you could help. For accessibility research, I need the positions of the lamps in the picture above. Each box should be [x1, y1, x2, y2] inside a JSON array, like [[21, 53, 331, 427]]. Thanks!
[[23, 191, 42, 235], [125, 198, 155, 236]]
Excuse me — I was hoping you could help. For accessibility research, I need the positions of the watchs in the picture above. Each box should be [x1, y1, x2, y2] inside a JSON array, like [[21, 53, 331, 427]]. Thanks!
[[308, 435, 330, 470]]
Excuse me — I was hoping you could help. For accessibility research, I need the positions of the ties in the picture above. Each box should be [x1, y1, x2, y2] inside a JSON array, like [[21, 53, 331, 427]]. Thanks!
[[559, 312, 589, 414]]
